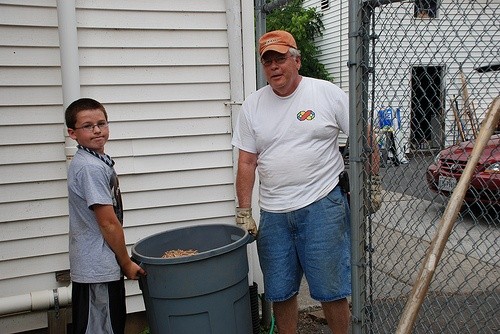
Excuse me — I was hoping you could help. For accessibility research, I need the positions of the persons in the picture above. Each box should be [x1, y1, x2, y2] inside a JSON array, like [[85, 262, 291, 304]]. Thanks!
[[230, 31, 384, 334], [63, 97, 146, 334]]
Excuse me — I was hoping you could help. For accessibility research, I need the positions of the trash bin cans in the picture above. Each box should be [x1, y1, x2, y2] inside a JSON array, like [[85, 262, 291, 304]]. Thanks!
[[125, 221, 258, 334]]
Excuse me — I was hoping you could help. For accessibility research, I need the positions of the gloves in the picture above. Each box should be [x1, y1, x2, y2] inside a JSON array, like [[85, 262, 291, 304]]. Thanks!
[[236, 206, 257, 237], [366, 175, 383, 215]]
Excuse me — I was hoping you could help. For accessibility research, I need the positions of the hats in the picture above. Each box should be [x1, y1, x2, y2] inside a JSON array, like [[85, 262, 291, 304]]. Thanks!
[[258, 30, 297, 60]]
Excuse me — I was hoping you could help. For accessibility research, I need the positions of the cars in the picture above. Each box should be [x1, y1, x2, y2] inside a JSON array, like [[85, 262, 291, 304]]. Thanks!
[[426, 134, 500, 204]]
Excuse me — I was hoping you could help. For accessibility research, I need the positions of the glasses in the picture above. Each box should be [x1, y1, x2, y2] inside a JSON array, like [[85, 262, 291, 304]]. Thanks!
[[261, 54, 294, 65], [76, 120, 109, 131]]
